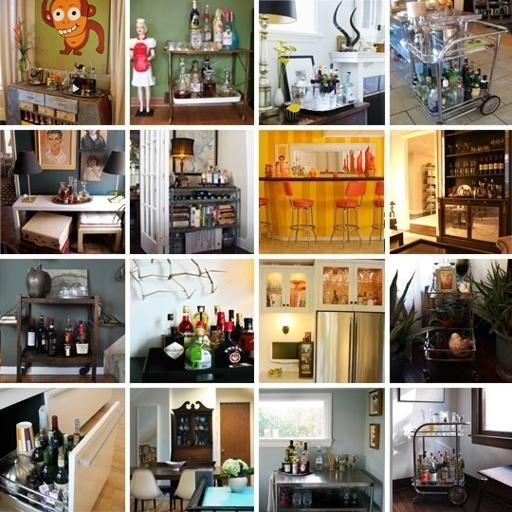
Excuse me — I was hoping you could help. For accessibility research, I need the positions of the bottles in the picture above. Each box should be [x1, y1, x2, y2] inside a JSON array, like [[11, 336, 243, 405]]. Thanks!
[[317, 62, 355, 106], [219, 70, 237, 97], [312, 450, 325, 471], [189, 60, 203, 99], [26, 317, 36, 350], [216, 312, 226, 342], [488, 156, 493, 174], [63, 335, 72, 358], [425, 67, 433, 84], [50, 415, 63, 457], [164, 326, 184, 363], [478, 157, 484, 174], [75, 324, 89, 356], [26, 478, 40, 507], [441, 58, 488, 106], [64, 435, 74, 469], [89, 70, 96, 94], [190, 0, 199, 29], [63, 190, 68, 203], [213, 13, 222, 51], [483, 157, 488, 174], [162, 314, 176, 336], [194, 305, 208, 329], [215, 322, 245, 367], [46, 431, 57, 459], [493, 155, 498, 174], [282, 440, 310, 474], [203, 75, 216, 97], [78, 182, 89, 201], [499, 154, 504, 174], [230, 312, 244, 344], [427, 89, 438, 113], [32, 437, 45, 479], [37, 316, 47, 353], [412, 72, 419, 89], [416, 448, 465, 485], [40, 452, 53, 491], [210, 305, 221, 336], [174, 66, 191, 99], [222, 12, 232, 50], [38, 428, 48, 448], [64, 317, 73, 335], [202, 13, 211, 41], [200, 164, 230, 185], [230, 11, 238, 49], [67, 186, 73, 203], [228, 309, 235, 325], [53, 454, 68, 492], [72, 419, 82, 446], [178, 306, 193, 348], [46, 318, 57, 357], [184, 329, 215, 372], [239, 317, 254, 363], [200, 55, 212, 79]]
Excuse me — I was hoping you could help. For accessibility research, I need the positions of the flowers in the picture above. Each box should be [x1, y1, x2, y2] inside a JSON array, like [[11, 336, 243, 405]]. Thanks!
[[11, 17, 33, 54], [221, 458, 254, 478], [273, 38, 298, 88]]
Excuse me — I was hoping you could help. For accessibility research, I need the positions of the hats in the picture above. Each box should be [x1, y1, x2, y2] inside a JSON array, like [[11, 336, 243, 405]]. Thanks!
[[137, 17, 145, 27]]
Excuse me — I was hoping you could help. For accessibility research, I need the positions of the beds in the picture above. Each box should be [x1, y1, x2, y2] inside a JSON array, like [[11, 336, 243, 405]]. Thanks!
[[8, 80, 111, 125]]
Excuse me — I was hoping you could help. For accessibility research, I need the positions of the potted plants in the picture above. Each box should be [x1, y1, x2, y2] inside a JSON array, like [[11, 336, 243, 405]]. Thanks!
[[389, 269, 444, 383], [467, 260, 512, 379]]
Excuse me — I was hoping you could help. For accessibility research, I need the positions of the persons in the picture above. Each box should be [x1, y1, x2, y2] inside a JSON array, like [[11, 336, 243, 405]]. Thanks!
[[38, 131, 72, 168], [129, 18, 156, 117]]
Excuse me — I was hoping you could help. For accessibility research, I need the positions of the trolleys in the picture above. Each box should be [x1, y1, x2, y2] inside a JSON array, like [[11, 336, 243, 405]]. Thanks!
[[422, 306, 479, 382], [409, 421, 472, 505], [399, 18, 509, 125]]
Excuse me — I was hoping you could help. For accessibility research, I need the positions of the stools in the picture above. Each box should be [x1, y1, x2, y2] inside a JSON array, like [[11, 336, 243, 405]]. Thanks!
[[20, 240, 71, 254], [77, 212, 122, 254], [259, 197, 273, 242], [475, 465, 512, 512]]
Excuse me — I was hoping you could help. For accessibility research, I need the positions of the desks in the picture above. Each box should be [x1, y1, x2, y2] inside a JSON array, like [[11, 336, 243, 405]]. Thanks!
[[330, 52, 385, 102], [261, 102, 370, 125]]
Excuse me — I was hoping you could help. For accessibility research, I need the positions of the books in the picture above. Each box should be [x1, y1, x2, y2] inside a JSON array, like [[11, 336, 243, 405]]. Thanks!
[[190, 205, 217, 228], [171, 206, 190, 228], [218, 204, 237, 225]]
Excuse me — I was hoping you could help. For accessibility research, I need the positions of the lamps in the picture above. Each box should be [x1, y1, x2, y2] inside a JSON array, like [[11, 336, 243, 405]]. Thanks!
[[102, 150, 125, 203], [12, 151, 42, 203], [259, 0, 297, 117], [170, 137, 194, 181]]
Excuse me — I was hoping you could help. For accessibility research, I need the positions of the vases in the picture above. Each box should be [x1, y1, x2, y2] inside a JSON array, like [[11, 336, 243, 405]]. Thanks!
[[274, 88, 284, 107], [18, 55, 29, 80], [227, 477, 247, 492]]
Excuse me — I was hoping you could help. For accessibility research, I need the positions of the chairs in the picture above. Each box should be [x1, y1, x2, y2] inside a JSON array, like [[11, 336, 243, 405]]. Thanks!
[[130, 469, 163, 512], [283, 182, 317, 249], [368, 182, 384, 242], [329, 182, 367, 247], [169, 468, 195, 512]]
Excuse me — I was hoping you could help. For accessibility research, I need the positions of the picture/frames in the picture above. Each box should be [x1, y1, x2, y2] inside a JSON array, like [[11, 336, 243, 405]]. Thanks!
[[280, 56, 320, 101], [173, 130, 217, 176], [368, 423, 379, 449], [42, 268, 88, 298], [34, 130, 77, 170], [399, 388, 445, 403], [368, 388, 382, 416]]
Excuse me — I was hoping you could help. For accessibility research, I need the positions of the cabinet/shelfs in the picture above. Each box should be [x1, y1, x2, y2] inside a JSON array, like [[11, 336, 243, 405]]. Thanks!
[[274, 470, 375, 512], [172, 401, 214, 461], [44, 388, 113, 435], [259, 265, 313, 313], [0, 392, 120, 511], [437, 130, 512, 254], [15, 294, 100, 382], [169, 186, 241, 254], [313, 259, 385, 312]]
